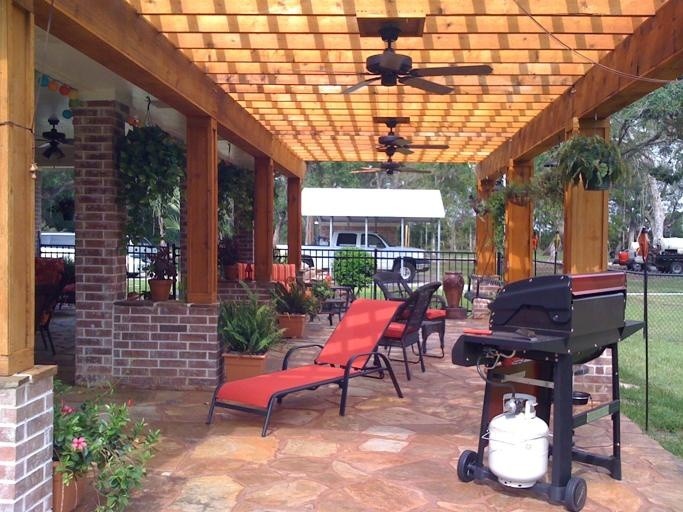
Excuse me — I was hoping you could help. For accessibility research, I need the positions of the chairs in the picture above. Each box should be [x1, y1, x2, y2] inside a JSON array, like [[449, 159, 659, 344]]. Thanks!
[[204, 262, 448, 440], [35, 256, 75, 355]]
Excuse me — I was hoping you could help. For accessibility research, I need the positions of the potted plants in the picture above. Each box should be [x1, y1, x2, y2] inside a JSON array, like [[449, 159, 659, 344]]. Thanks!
[[486, 134, 640, 207]]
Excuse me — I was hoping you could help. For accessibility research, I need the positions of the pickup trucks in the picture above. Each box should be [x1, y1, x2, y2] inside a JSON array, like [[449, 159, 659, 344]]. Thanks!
[[275, 230, 431, 283]]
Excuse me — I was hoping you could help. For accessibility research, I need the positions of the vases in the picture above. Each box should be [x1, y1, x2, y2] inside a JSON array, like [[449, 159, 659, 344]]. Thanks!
[[148, 278, 173, 302], [53, 460, 85, 511], [442, 271, 467, 319]]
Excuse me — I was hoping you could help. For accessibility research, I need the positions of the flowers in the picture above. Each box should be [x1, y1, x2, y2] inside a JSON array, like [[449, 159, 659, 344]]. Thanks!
[[53, 369, 166, 512], [140, 246, 178, 280]]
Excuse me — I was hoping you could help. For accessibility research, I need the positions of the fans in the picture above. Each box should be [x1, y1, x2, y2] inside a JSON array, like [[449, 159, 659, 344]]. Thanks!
[[349, 116, 450, 175], [35, 118, 74, 160], [336, 16, 493, 93]]
